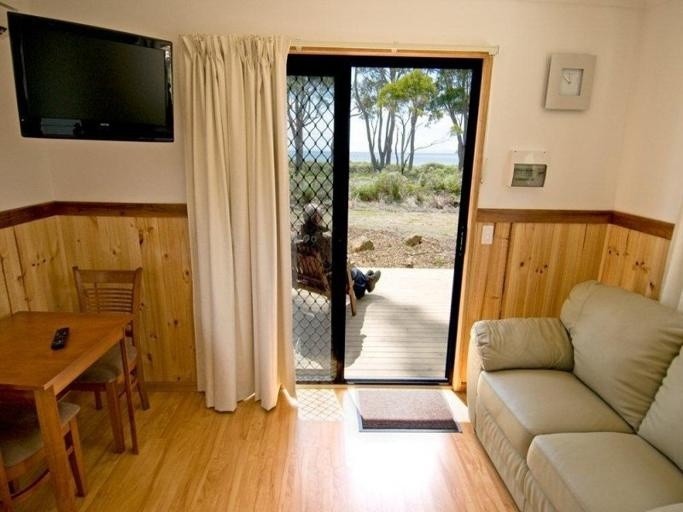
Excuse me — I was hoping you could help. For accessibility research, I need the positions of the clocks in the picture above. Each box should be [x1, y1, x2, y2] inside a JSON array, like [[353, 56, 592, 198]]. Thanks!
[[544, 51, 598, 112]]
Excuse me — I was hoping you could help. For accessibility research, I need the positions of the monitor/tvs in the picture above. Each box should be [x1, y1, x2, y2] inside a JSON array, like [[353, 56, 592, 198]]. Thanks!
[[6, 11, 175, 143]]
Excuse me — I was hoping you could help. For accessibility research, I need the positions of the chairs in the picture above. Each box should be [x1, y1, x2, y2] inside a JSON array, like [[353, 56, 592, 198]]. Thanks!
[[0, 263, 144, 512], [289, 228, 358, 317]]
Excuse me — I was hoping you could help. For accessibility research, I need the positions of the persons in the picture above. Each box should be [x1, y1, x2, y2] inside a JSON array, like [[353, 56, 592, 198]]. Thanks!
[[296, 204, 381, 299]]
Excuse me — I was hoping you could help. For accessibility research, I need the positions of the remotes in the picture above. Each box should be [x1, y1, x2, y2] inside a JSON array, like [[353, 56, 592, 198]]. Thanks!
[[51, 327, 69, 350]]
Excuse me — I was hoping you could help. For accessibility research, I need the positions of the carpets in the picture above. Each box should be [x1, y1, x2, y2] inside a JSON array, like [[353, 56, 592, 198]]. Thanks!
[[351, 386, 463, 436]]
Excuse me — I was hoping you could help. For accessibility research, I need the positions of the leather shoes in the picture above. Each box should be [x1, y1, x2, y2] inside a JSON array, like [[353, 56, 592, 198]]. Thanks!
[[366, 270, 380, 292], [365, 270, 374, 276]]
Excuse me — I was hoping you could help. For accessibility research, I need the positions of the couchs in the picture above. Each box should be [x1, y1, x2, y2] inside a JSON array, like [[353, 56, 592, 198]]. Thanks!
[[468, 278, 683, 512]]
